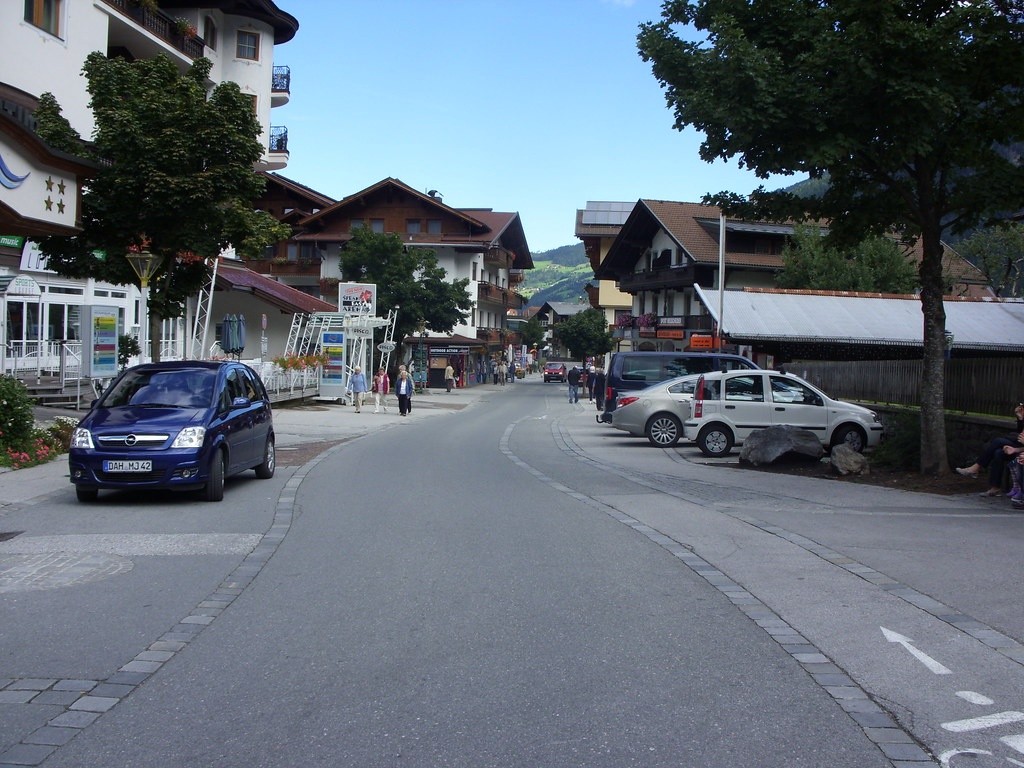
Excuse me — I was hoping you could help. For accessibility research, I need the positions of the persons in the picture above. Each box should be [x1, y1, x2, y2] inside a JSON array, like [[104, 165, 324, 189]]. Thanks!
[[373, 368, 390, 413], [539, 366, 544, 377], [586, 366, 596, 404], [568, 366, 581, 403], [955, 405, 1024, 508], [444, 363, 454, 392], [395, 371, 412, 416], [348, 366, 367, 413], [494, 361, 508, 386], [397, 365, 415, 414], [593, 369, 605, 410], [509, 362, 516, 384]]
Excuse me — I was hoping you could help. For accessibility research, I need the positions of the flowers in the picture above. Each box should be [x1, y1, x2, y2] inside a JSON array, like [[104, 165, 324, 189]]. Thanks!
[[510, 251, 516, 260], [615, 311, 635, 328], [173, 16, 198, 41], [273, 350, 332, 371], [480, 328, 495, 343], [483, 284, 496, 296], [637, 312, 658, 328], [502, 287, 515, 300], [321, 277, 338, 291], [500, 329, 516, 343]]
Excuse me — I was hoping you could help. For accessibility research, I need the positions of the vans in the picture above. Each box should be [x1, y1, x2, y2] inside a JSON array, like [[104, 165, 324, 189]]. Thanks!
[[595, 351, 791, 425]]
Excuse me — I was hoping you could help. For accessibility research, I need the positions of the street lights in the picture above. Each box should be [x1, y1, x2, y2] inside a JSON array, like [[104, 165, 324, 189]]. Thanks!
[[125, 241, 165, 364], [416, 319, 427, 393], [533, 342, 538, 364]]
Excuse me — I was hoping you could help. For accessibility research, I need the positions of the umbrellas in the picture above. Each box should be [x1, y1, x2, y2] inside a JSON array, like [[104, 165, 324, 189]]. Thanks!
[[220, 313, 246, 359]]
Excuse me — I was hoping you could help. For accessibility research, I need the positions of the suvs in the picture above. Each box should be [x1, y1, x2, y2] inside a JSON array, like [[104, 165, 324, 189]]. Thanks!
[[544, 362, 568, 383]]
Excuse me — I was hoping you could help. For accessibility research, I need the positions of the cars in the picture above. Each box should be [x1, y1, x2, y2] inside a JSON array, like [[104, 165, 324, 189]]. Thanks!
[[684, 370, 885, 457], [610, 373, 798, 449], [576, 367, 590, 386], [67, 359, 275, 501]]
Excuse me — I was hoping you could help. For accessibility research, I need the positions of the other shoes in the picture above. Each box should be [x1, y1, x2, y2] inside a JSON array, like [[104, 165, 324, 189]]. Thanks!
[[373, 411, 380, 414], [1006, 487, 1021, 497], [408, 411, 411, 415], [1011, 492, 1024, 501], [956, 467, 979, 479], [384, 409, 387, 415], [1012, 501, 1024, 510], [590, 400, 595, 404], [400, 411, 406, 416], [355, 410, 360, 413], [598, 408, 604, 411], [979, 488, 1003, 497]]
